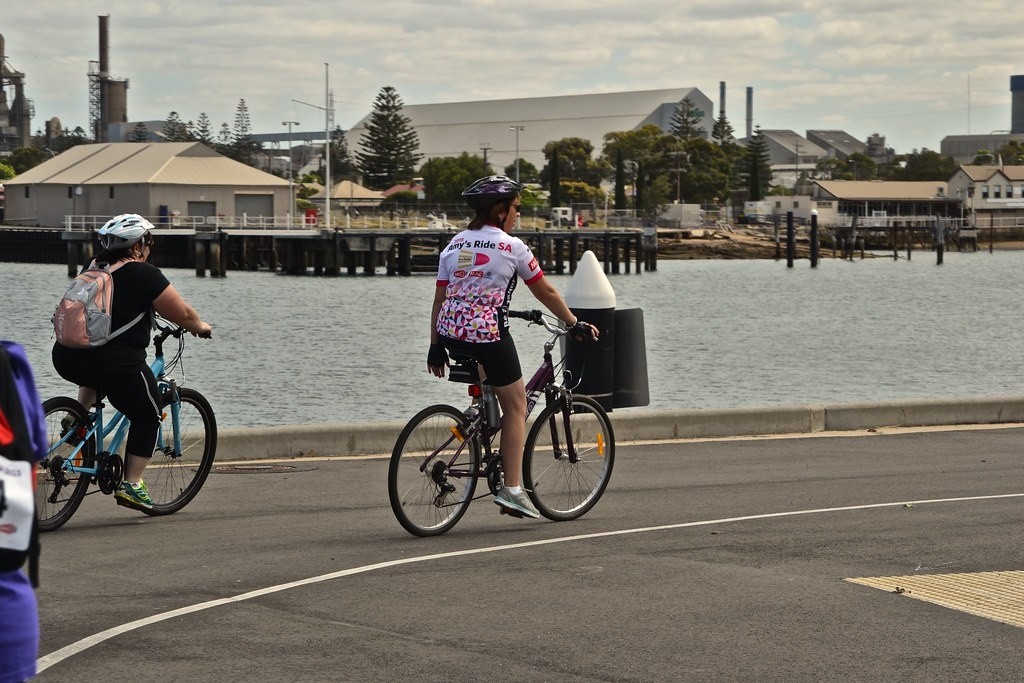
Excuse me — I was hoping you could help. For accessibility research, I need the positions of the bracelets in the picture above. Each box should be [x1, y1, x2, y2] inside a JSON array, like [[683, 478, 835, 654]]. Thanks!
[[564, 317, 577, 329]]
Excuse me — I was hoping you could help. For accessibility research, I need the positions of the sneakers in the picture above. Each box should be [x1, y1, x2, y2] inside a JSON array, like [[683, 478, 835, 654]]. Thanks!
[[113, 478, 156, 511], [59, 414, 76, 433], [463, 402, 479, 419], [493, 487, 542, 519]]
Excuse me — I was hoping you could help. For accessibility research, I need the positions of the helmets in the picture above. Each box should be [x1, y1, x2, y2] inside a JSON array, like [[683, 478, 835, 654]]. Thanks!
[[97, 213, 156, 251], [460, 175, 528, 209]]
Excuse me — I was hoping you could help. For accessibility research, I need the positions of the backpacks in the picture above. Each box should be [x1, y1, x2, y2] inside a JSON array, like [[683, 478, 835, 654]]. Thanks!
[[0, 337, 42, 588], [51, 255, 148, 350]]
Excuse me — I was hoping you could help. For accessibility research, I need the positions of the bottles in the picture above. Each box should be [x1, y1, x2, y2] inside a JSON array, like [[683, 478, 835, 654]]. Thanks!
[[482, 378, 501, 428]]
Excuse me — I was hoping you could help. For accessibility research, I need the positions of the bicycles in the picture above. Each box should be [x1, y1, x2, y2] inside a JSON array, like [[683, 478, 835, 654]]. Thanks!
[[37, 314, 219, 534], [387, 308, 616, 539]]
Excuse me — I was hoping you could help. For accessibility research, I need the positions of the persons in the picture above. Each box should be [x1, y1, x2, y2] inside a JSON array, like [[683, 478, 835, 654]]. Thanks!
[[0, 339, 48, 683], [427, 174, 600, 518], [51, 213, 212, 509]]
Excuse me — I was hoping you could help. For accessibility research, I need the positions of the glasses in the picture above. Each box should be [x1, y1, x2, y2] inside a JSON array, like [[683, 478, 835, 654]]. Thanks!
[[138, 240, 157, 249], [502, 204, 521, 212]]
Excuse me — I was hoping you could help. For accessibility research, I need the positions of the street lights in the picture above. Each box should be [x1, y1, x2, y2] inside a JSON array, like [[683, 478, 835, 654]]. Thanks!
[[282, 120, 301, 228], [509, 125, 525, 230]]
